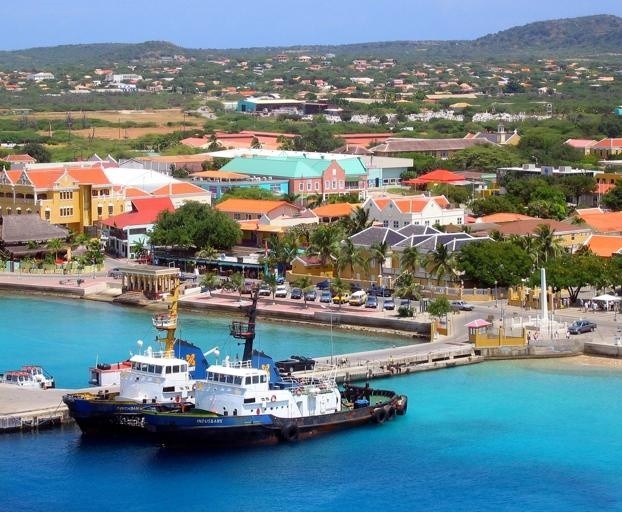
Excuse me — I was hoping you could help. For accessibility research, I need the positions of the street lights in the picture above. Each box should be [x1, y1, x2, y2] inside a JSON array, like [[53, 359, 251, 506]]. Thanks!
[[493, 279, 499, 307]]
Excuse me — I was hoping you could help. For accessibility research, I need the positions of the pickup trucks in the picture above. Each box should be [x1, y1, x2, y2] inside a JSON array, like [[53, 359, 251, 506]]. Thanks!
[[273, 353, 319, 372]]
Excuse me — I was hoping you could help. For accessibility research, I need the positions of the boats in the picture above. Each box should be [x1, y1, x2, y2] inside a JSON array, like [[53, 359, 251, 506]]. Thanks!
[[0, 370, 43, 391], [20, 365, 58, 389], [145, 281, 409, 455], [60, 282, 223, 441]]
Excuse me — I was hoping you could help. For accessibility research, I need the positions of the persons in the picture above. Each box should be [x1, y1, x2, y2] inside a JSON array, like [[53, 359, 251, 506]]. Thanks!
[[558, 297, 622, 314], [527, 330, 570, 342]]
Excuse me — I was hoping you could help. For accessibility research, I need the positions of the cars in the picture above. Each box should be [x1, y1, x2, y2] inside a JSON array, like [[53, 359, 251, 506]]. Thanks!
[[567, 319, 596, 336], [257, 276, 413, 314], [107, 266, 121, 278], [450, 299, 474, 312]]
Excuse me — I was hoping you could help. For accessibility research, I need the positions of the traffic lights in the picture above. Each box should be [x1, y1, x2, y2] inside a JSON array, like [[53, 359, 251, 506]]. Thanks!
[[552, 286, 557, 293]]
